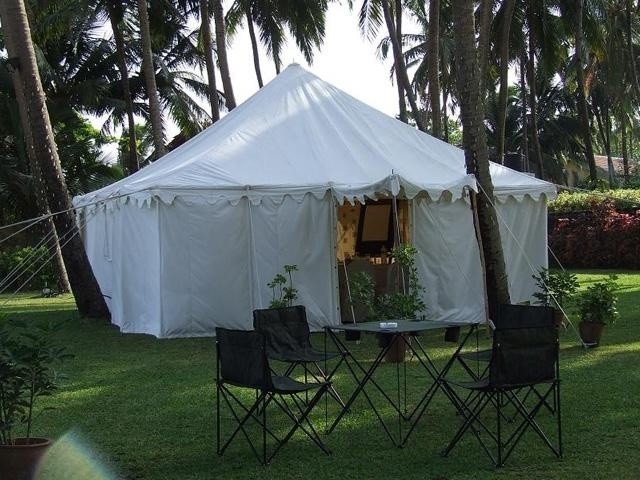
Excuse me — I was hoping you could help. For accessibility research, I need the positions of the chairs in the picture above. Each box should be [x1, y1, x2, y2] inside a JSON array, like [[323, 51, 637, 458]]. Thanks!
[[212, 325, 334, 469], [440, 305, 564, 469], [253, 306, 342, 409]]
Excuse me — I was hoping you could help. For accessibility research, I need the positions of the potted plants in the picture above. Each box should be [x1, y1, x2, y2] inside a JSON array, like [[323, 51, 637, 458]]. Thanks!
[[575, 271, 621, 349], [0, 309, 74, 480], [353, 245, 425, 361]]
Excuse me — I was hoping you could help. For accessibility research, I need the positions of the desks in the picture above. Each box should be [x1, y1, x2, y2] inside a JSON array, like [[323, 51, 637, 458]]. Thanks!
[[323, 317, 480, 448]]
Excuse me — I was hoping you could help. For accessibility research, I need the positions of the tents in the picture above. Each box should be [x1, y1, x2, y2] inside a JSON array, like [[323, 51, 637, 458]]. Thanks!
[[69, 60, 559, 340]]
